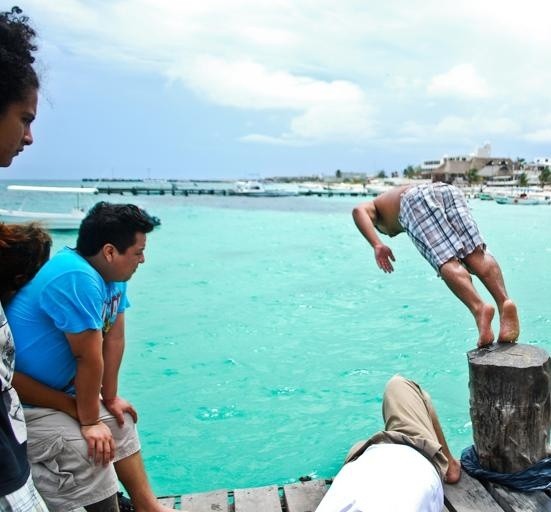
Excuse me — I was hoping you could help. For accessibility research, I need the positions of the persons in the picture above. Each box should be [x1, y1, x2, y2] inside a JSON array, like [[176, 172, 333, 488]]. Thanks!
[[2, 14, 48, 512], [315, 376, 460, 512], [3, 201, 181, 512], [352, 182, 519, 347], [1, 222, 81, 421]]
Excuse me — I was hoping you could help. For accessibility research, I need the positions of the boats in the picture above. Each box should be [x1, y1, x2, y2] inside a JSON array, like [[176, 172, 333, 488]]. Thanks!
[[298, 177, 433, 194], [0, 185, 98, 229], [474, 192, 551, 205], [234, 181, 264, 193]]
